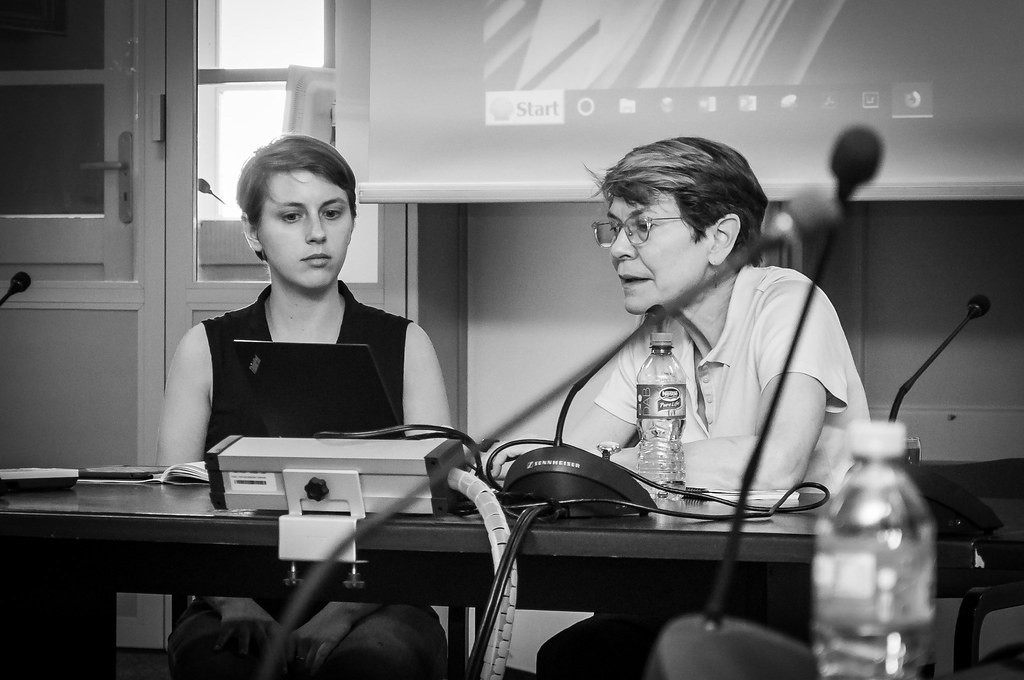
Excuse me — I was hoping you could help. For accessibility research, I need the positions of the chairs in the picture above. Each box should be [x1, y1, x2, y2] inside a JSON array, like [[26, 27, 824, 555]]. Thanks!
[[912, 457, 1024, 670]]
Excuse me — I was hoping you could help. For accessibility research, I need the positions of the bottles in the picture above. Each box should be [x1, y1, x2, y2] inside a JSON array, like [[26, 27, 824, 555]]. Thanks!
[[809, 420, 938, 680], [636, 333, 686, 503]]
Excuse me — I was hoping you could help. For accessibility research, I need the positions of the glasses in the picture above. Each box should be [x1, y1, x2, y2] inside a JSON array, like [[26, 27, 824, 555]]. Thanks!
[[592, 215, 683, 249]]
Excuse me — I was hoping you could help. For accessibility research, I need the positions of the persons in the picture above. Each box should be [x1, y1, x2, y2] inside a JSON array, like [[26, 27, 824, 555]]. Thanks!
[[469, 138, 869, 680], [154, 136, 467, 680]]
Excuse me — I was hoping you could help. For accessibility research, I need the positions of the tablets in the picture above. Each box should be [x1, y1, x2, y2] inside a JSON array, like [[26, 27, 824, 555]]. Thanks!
[[79, 464, 173, 481]]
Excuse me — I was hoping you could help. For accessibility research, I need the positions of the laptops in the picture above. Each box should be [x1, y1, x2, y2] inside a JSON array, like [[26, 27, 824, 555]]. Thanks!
[[235, 338, 406, 440]]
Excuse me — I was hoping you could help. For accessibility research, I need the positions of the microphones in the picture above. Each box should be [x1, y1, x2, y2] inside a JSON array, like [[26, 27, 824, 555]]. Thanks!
[[265, 125, 1003, 680], [0, 271, 32, 308]]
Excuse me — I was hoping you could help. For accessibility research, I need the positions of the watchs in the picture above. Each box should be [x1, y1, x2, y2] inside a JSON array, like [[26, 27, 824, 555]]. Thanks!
[[597, 440, 620, 460]]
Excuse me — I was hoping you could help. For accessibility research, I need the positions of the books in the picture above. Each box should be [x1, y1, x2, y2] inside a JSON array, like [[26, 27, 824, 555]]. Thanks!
[[77, 461, 207, 487]]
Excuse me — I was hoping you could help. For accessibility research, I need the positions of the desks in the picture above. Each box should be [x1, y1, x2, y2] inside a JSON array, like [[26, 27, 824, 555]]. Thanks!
[[0, 480, 991, 680]]
